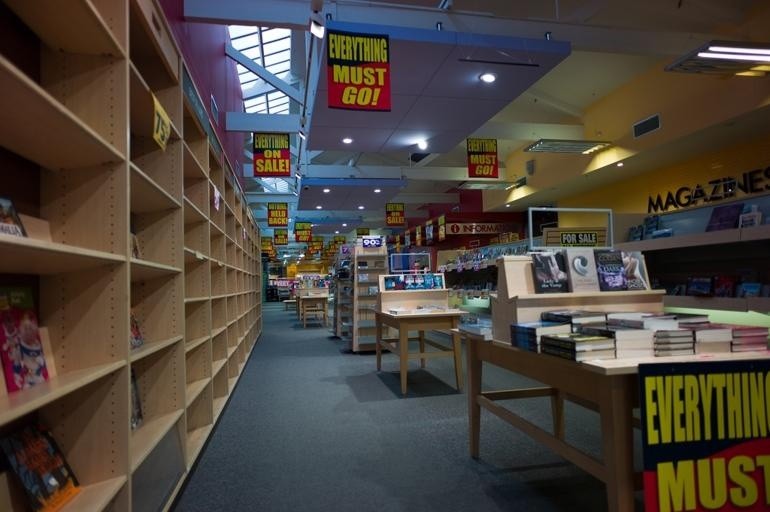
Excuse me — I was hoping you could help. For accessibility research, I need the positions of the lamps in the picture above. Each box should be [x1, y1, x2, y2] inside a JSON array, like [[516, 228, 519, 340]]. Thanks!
[[523, 139, 610, 155], [299, 127, 306, 140], [309, 12, 325, 39], [665, 40, 770, 76], [295, 170, 302, 179], [456, 182, 519, 190]]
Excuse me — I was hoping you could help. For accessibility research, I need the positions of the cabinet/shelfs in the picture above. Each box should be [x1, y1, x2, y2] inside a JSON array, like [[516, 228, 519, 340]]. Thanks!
[[435, 195, 770, 340], [0, 0, 262, 512], [333, 245, 390, 352]]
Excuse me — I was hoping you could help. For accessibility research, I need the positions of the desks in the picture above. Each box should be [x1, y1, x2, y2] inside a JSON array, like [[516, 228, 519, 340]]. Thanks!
[[282, 287, 331, 329], [458, 325, 770, 511], [374, 308, 470, 395]]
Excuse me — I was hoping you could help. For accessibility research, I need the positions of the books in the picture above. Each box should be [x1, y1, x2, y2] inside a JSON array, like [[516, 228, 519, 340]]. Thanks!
[[131, 368, 144, 432], [529, 247, 648, 294], [0, 193, 27, 237], [0, 288, 50, 393], [0, 410, 83, 511], [130, 313, 143, 349]]
